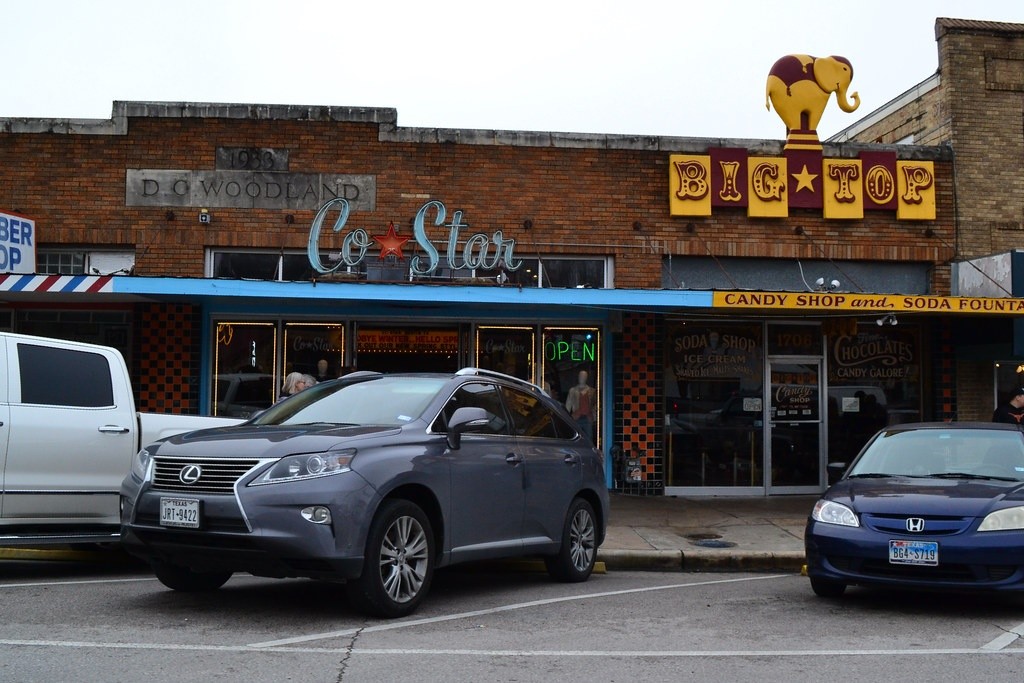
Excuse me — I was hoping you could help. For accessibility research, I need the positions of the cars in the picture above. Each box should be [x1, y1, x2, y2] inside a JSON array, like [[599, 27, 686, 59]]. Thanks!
[[805, 419, 1024, 604], [669, 385, 922, 456], [207, 367, 299, 420]]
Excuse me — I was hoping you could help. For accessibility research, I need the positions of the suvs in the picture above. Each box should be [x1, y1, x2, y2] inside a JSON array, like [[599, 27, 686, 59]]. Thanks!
[[118, 368, 610, 622]]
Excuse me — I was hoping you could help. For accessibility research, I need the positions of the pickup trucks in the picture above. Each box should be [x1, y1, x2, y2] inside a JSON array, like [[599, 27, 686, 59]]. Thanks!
[[0, 332, 252, 544]]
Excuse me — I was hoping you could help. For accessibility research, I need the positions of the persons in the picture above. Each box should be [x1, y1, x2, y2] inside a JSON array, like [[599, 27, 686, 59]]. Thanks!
[[722, 386, 888, 485], [314, 359, 332, 383], [276, 372, 317, 402], [564, 371, 597, 443], [992, 385, 1024, 424]]
